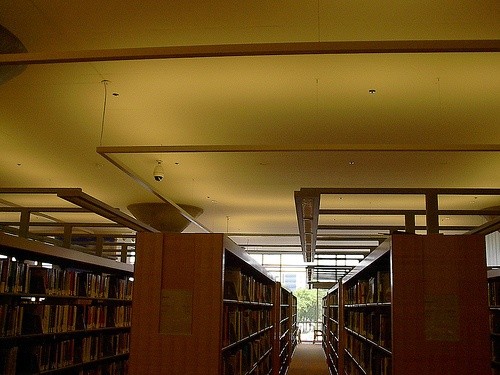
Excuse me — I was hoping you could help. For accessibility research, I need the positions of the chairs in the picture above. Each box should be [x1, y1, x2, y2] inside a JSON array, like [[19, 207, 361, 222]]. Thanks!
[[313, 330, 322, 343]]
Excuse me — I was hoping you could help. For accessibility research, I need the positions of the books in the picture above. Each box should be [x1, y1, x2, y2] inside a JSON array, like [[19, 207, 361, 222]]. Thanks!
[[0, 260, 134, 375], [223, 270, 296, 375], [324, 272, 392, 375], [488, 281, 500, 362]]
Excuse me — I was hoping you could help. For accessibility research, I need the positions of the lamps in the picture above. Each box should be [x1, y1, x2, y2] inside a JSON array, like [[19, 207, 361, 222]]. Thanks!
[[294, 190, 320, 262], [56, 190, 158, 232]]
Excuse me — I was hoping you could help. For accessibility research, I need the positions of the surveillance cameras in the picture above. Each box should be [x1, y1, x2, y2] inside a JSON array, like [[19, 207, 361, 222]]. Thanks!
[[153, 165, 163, 181]]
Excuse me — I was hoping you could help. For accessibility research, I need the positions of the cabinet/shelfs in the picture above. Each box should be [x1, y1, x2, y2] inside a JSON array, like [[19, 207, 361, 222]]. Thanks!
[[341, 234, 493, 375], [322, 292, 328, 363], [487, 268, 500, 375], [328, 282, 341, 375], [0, 232, 133, 375], [128, 233, 297, 375]]
